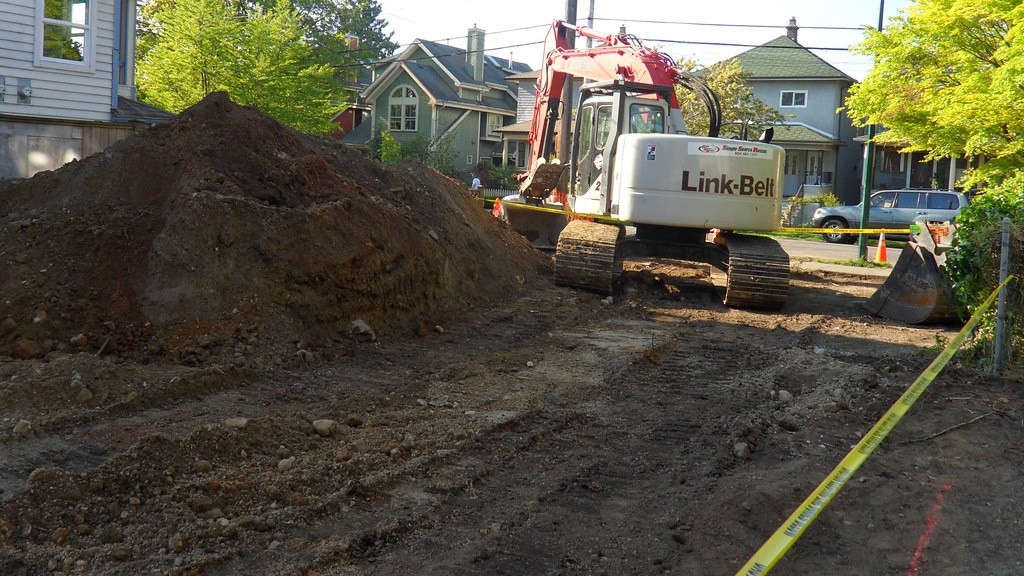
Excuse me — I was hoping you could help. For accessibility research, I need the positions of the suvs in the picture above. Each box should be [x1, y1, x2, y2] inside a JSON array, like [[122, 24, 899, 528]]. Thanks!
[[811, 188, 970, 246]]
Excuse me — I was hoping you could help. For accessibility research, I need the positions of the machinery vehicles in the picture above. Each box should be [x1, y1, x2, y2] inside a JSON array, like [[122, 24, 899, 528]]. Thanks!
[[501, 19, 791, 311]]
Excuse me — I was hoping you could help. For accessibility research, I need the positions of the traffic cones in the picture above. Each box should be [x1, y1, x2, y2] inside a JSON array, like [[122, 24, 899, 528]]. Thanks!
[[873, 232, 887, 262]]
